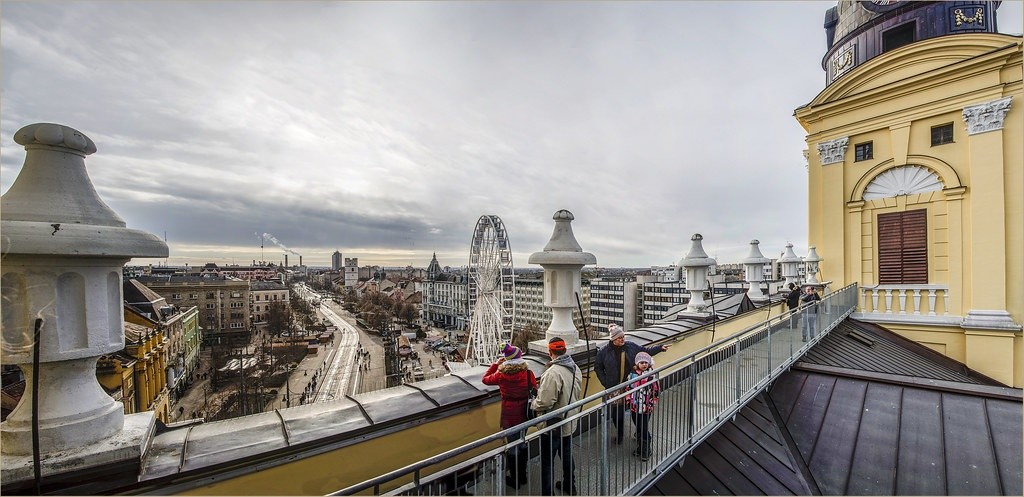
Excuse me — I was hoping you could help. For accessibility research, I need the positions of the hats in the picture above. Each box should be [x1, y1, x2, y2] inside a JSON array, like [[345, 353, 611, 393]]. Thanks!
[[608, 323, 624, 341], [547, 337, 565, 352], [635, 351, 651, 365], [499, 342, 522, 360]]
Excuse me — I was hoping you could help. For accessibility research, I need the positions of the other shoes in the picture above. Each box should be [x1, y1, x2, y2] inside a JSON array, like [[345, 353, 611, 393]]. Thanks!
[[553, 481, 578, 496], [505, 472, 528, 491], [611, 430, 653, 461]]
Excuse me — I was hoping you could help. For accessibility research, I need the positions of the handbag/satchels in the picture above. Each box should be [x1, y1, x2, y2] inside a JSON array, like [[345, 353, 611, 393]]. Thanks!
[[546, 416, 564, 436], [602, 385, 625, 406], [526, 392, 538, 427]]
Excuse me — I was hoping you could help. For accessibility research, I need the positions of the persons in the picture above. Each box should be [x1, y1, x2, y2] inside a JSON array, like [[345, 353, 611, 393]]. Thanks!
[[300, 341, 372, 406], [595, 322, 675, 447], [622, 350, 661, 461], [800, 287, 820, 341], [786, 283, 799, 330], [481, 342, 539, 492], [530, 337, 582, 496]]
